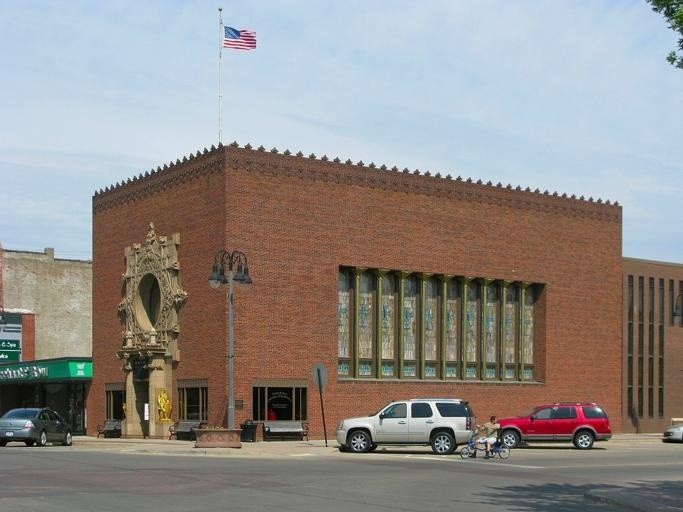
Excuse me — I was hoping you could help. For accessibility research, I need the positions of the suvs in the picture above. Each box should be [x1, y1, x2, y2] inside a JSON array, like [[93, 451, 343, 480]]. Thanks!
[[336, 397, 477, 454], [492, 402, 612, 450]]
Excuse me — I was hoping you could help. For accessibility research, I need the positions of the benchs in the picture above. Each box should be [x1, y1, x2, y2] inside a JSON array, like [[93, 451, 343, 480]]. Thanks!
[[96, 419, 121, 438], [248, 421, 306, 441], [168, 421, 202, 440]]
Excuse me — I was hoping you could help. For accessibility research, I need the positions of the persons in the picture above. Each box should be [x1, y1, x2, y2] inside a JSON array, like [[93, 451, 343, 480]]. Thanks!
[[473, 416, 500, 456]]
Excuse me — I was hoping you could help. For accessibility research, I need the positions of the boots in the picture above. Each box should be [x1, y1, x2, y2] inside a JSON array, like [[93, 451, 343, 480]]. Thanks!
[[471, 452, 476, 458], [484, 451, 489, 459]]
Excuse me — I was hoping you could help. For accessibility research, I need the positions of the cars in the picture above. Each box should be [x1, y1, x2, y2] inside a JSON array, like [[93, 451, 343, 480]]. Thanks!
[[661, 423, 682, 444], [0, 407, 72, 447]]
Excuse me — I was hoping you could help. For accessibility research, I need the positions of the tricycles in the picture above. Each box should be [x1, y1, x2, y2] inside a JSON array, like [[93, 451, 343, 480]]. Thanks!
[[459, 430, 511, 459]]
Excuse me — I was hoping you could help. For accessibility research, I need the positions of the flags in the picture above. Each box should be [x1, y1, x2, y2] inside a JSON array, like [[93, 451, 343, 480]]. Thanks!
[[223, 28, 256, 51]]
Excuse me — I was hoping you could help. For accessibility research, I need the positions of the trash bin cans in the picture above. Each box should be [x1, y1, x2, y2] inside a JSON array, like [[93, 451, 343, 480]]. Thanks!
[[239, 419, 258, 442]]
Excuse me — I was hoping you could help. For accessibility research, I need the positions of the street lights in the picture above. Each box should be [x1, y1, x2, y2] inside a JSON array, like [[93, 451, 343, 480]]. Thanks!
[[208, 250, 252, 429]]
